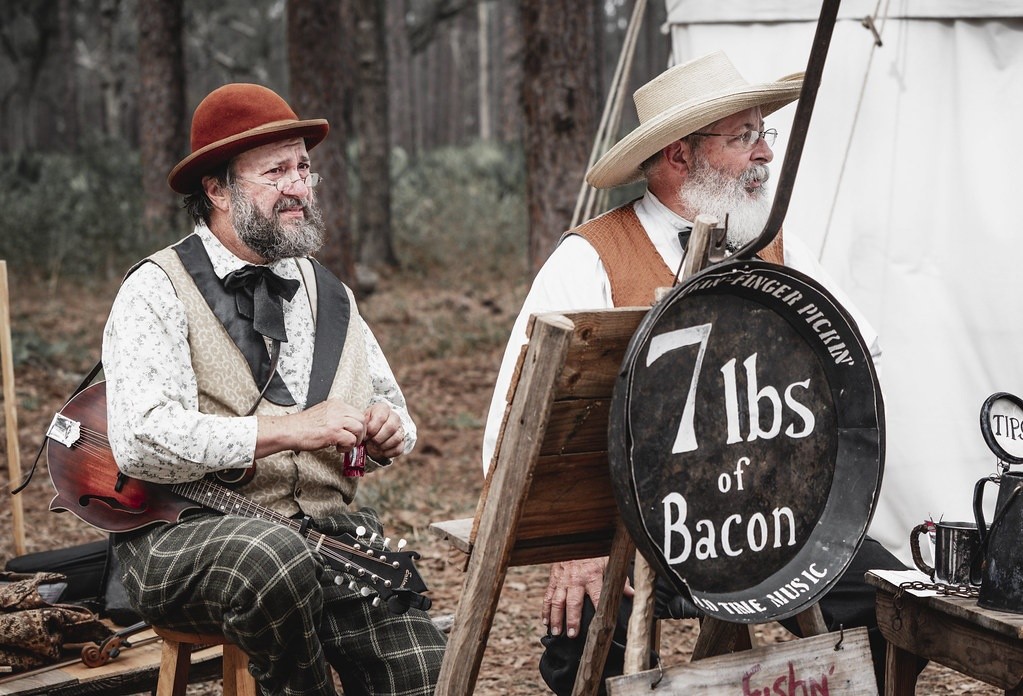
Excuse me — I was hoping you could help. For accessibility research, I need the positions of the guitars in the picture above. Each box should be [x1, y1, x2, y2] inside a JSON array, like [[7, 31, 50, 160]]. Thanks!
[[42, 375, 438, 618]]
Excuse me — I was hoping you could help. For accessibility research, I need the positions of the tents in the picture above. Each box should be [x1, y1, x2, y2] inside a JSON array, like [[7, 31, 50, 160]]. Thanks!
[[568, 0, 1023, 571]]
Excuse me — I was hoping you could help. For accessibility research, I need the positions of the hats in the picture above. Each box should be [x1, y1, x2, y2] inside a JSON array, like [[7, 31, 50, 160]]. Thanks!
[[167, 82, 329, 193], [584, 50, 806, 190]]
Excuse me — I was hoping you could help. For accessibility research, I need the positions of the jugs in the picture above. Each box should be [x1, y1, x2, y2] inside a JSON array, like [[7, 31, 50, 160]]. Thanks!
[[972, 391, 1023, 614]]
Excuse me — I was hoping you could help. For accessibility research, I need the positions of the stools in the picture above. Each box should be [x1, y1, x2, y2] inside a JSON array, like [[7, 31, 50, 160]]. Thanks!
[[149, 623, 256, 696]]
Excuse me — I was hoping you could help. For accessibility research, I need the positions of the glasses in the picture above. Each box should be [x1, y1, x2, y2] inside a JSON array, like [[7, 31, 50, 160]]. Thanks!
[[236, 172, 326, 193], [690, 128, 778, 150]]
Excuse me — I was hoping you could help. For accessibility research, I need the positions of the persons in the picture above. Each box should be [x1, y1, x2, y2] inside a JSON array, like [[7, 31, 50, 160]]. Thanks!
[[99, 82, 448, 696], [482, 47, 930, 696]]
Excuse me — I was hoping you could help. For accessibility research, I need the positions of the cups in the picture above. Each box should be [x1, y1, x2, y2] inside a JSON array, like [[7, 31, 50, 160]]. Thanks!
[[910, 522, 992, 589]]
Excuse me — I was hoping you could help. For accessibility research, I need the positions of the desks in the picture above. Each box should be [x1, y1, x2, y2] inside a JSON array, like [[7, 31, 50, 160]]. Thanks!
[[864, 570, 1023, 696]]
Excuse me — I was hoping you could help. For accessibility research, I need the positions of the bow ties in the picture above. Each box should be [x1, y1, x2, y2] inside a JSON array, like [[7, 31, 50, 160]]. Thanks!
[[678, 229, 740, 252], [223, 264, 300, 343]]
